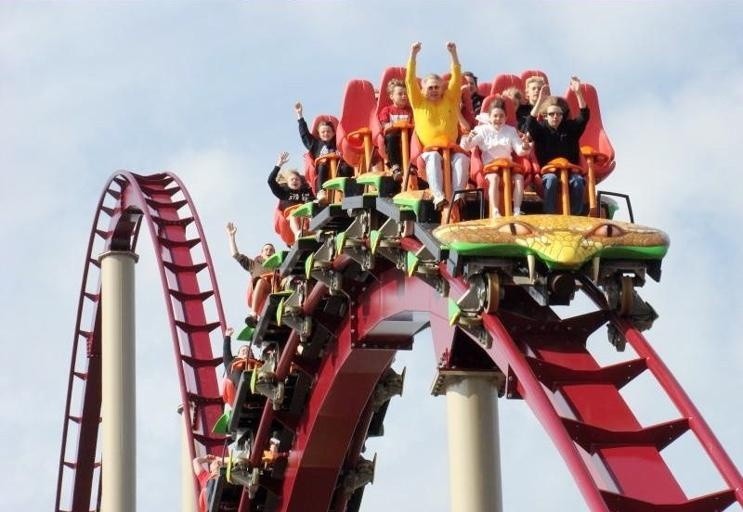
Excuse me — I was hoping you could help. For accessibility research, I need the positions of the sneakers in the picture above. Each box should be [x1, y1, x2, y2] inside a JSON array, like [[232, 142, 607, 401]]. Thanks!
[[434, 196, 448, 211], [317, 190, 328, 205], [245, 314, 257, 328], [454, 193, 466, 205], [393, 169, 401, 181], [409, 167, 417, 174], [295, 229, 307, 241]]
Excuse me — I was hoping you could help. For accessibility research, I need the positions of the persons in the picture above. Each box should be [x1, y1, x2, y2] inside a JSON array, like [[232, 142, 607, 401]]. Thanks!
[[504, 88, 524, 102], [293, 100, 354, 205], [378, 79, 413, 179], [462, 69, 486, 115], [524, 74, 592, 214], [460, 100, 533, 220], [404, 40, 469, 213], [267, 152, 315, 240], [226, 222, 276, 330], [222, 324, 255, 389], [518, 75, 548, 134], [191, 453, 228, 512]]
